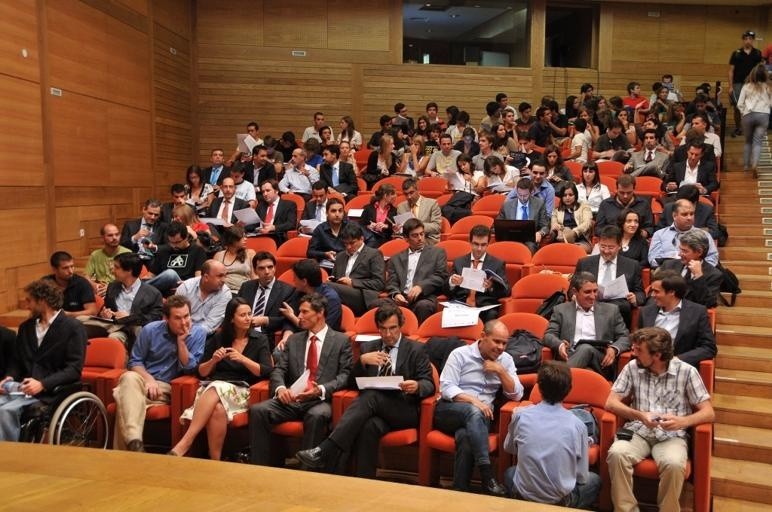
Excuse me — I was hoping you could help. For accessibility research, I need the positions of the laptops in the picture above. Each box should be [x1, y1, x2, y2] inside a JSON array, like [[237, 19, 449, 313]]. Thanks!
[[494, 220, 536, 243]]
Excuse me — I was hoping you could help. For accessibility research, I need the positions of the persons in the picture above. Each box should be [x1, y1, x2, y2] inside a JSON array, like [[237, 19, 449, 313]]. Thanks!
[[267, 256, 347, 366], [245, 292, 354, 466], [734, 64, 772, 175], [636, 268, 719, 368], [498, 358, 602, 512], [760, 41, 772, 132], [434, 319, 525, 498], [540, 271, 631, 382], [78, 74, 723, 340], [606, 326, 716, 512], [164, 296, 275, 463], [296, 303, 435, 476], [1, 278, 87, 441], [728, 30, 763, 136], [110, 294, 208, 456], [40, 249, 100, 322]]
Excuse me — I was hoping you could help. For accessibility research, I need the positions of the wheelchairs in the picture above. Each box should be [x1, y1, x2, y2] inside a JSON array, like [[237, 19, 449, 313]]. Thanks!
[[18, 340, 113, 450]]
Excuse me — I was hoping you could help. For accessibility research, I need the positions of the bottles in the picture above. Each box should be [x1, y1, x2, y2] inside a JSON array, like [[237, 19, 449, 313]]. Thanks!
[[3, 380, 26, 396]]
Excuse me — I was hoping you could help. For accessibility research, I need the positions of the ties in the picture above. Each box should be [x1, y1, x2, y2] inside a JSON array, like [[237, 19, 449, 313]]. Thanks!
[[466, 260, 479, 306], [379, 346, 393, 376], [646, 152, 652, 161], [684, 267, 691, 281], [211, 169, 218, 185], [304, 336, 317, 392], [316, 168, 338, 221], [253, 286, 268, 316], [522, 206, 527, 220], [222, 201, 273, 223], [602, 261, 612, 282]]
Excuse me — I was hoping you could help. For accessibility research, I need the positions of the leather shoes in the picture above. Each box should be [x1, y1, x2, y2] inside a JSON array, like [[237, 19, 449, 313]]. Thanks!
[[482, 479, 508, 496], [295, 447, 326, 467]]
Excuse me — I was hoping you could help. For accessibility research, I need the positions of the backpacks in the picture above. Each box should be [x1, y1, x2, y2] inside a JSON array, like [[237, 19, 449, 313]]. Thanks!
[[568, 404, 600, 444], [505, 329, 543, 374], [537, 288, 565, 320], [717, 263, 741, 306]]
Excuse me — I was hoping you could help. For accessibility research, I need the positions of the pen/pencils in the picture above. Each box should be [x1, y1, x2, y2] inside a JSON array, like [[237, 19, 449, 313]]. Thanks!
[[381, 350, 392, 364]]
